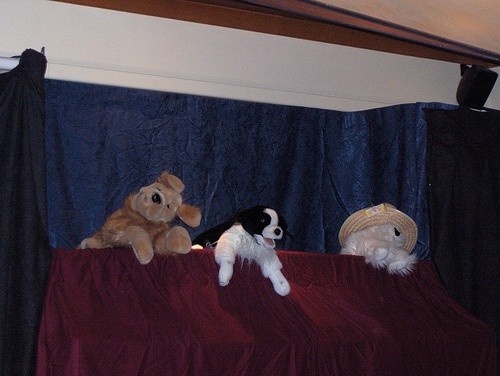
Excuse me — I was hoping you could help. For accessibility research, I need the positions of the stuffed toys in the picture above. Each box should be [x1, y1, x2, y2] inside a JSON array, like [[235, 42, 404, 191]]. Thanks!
[[338, 203, 418, 274], [76, 171, 203, 264], [189, 203, 293, 297]]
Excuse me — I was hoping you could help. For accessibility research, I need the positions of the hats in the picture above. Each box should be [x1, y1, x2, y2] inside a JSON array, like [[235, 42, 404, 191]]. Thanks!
[[332, 202, 417, 254]]
